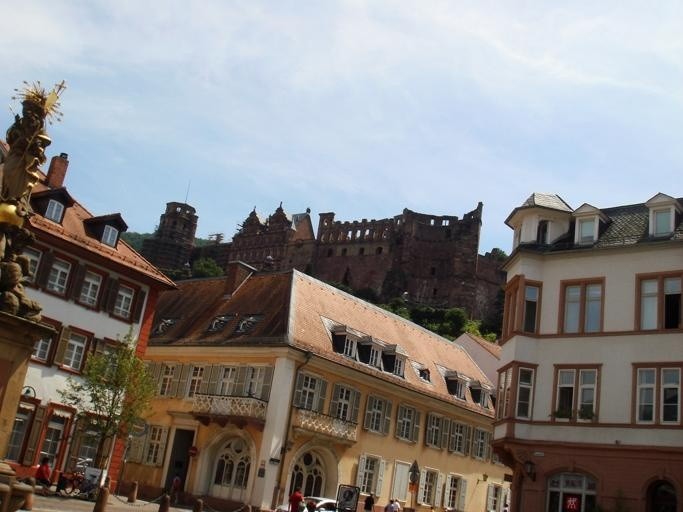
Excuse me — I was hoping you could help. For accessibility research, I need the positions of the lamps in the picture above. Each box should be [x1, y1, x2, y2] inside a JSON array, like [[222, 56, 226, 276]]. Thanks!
[[523, 458, 539, 483], [22, 385, 37, 405]]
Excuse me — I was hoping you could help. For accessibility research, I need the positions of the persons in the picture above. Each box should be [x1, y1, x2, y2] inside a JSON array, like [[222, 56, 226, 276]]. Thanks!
[[169, 472, 181, 505], [306, 500, 316, 511], [394, 498, 401, 512], [362, 491, 377, 511], [384, 499, 395, 512], [33, 456, 53, 489], [287, 485, 305, 512]]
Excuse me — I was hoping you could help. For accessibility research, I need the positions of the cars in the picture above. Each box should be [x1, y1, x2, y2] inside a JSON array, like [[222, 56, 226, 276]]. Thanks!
[[272, 492, 339, 512], [73, 453, 101, 481]]
